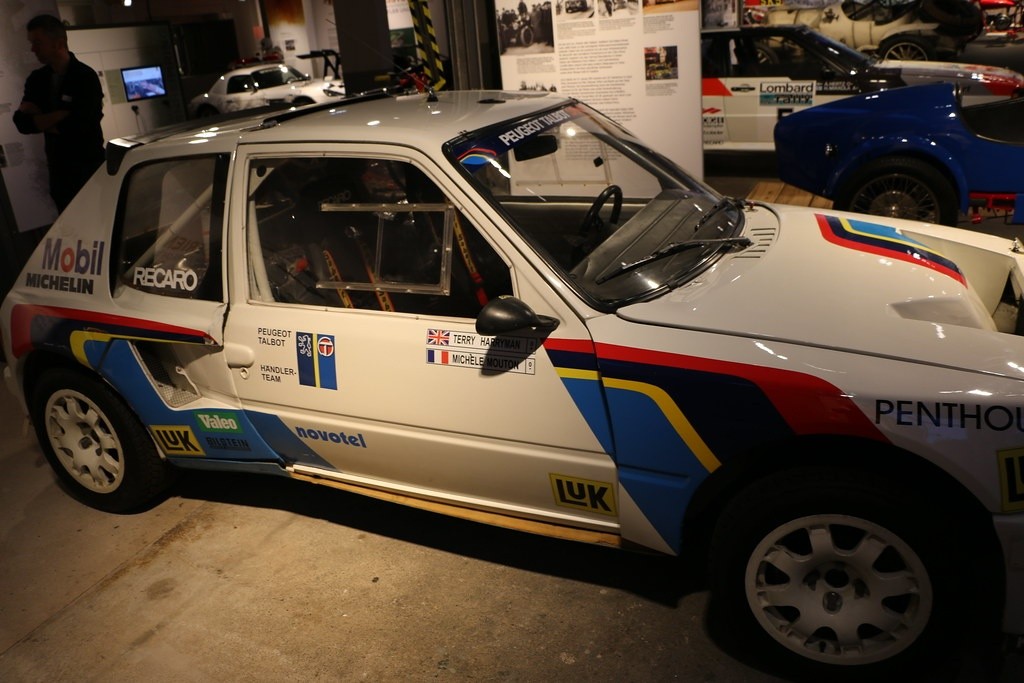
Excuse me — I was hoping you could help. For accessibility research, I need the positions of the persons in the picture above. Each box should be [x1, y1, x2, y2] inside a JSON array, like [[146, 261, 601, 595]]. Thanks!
[[12, 14, 105, 214]]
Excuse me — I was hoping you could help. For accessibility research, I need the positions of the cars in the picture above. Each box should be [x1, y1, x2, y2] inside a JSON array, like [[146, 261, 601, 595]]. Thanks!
[[0, 89, 1024, 683], [191, 63, 350, 114], [698, 22, 1024, 241]]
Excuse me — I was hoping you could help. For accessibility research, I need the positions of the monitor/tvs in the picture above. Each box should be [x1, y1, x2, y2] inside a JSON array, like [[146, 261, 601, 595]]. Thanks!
[[121, 64, 168, 102]]
